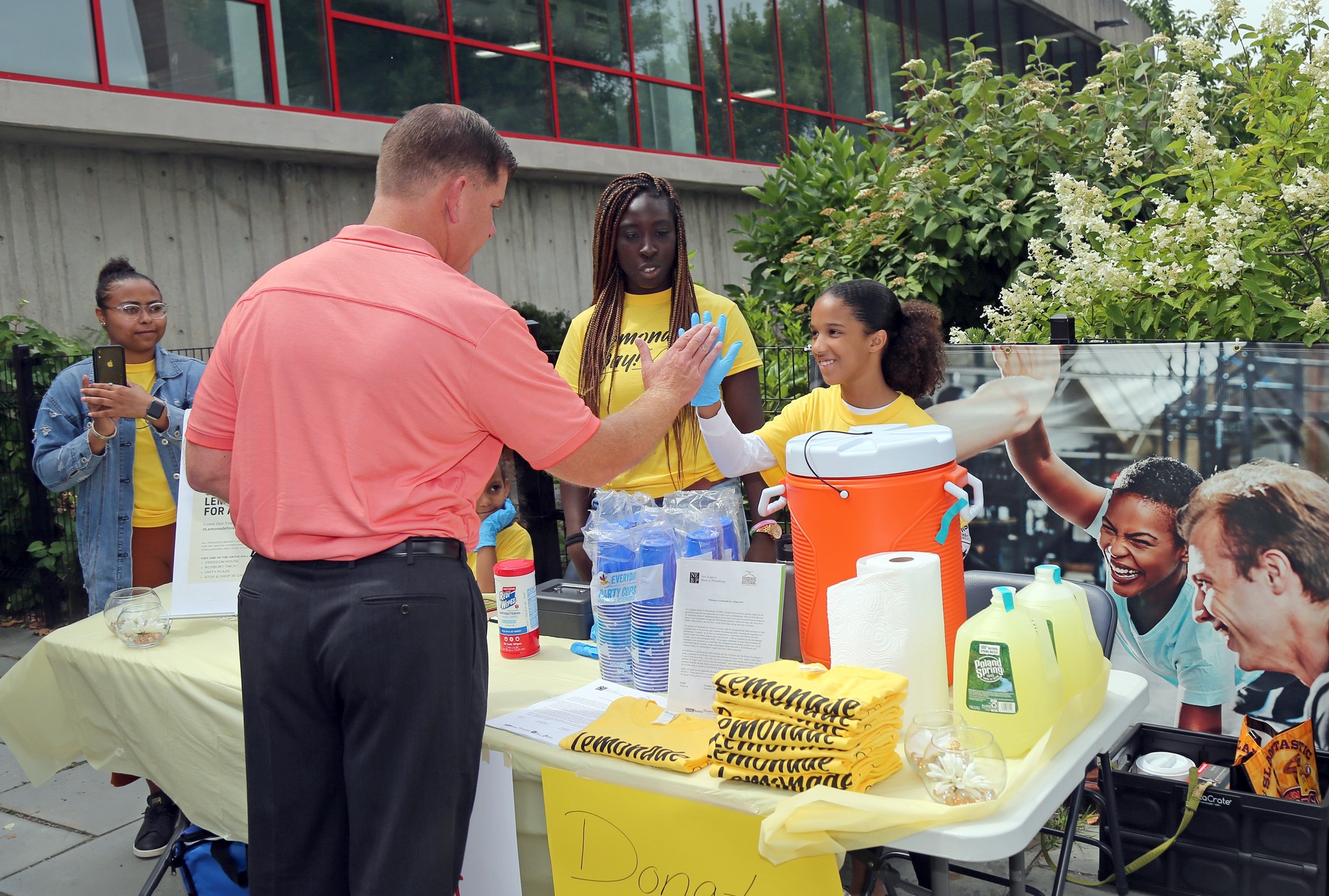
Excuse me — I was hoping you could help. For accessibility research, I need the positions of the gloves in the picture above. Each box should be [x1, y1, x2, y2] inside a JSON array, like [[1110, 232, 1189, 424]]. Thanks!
[[677, 311, 742, 407], [473, 498, 516, 552]]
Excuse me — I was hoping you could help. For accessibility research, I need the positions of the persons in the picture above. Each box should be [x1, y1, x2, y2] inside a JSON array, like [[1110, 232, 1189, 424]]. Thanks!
[[33, 259, 208, 858], [467, 461, 534, 593], [679, 279, 972, 896], [186, 102, 723, 896], [551, 172, 782, 581], [924, 343, 1329, 772]]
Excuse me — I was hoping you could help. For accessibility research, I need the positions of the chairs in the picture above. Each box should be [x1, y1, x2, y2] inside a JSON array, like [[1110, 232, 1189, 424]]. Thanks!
[[855, 569, 1120, 896]]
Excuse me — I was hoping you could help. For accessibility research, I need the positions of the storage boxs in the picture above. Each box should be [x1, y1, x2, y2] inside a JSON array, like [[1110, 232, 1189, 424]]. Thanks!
[[1099, 722, 1329, 896]]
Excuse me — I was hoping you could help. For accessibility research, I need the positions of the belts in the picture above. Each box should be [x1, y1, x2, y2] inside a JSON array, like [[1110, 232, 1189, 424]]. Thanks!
[[363, 541, 468, 561]]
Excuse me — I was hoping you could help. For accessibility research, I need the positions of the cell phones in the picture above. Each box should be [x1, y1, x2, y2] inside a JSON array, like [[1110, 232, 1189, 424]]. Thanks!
[[93, 345, 127, 388]]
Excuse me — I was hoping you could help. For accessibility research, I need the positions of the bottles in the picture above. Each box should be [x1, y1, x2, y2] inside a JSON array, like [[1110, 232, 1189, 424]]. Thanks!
[[923, 726, 1007, 807], [103, 587, 161, 636], [117, 602, 169, 648], [1016, 564, 1111, 753], [949, 586, 1058, 758], [493, 558, 541, 659], [904, 709, 972, 776]]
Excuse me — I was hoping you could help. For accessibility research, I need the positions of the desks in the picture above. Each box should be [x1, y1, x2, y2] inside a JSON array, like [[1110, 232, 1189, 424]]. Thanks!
[[0, 576, 1147, 895]]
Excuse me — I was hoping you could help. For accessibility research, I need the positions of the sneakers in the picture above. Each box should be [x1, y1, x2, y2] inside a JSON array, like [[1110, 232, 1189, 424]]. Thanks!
[[133, 791, 189, 857]]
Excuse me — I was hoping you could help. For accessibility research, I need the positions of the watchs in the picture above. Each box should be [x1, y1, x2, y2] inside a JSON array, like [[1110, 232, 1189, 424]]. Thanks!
[[754, 524, 782, 540], [144, 396, 165, 422]]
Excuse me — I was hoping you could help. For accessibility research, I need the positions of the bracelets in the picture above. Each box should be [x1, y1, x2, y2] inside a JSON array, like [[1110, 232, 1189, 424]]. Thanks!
[[749, 519, 777, 538], [91, 420, 117, 439], [565, 533, 584, 546]]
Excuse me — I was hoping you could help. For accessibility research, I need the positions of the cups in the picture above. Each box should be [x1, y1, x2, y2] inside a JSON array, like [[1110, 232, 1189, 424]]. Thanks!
[[596, 512, 741, 692]]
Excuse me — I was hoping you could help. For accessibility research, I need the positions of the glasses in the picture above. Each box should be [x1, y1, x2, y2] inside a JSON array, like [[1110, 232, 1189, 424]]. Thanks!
[[100, 303, 170, 321]]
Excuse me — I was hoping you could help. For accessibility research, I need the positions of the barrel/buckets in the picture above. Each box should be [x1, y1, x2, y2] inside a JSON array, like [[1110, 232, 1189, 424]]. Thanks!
[[786, 424, 984, 685]]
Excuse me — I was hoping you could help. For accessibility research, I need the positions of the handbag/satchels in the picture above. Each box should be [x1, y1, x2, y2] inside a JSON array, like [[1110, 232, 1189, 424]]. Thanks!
[[1229, 713, 1323, 805], [164, 824, 248, 896]]
